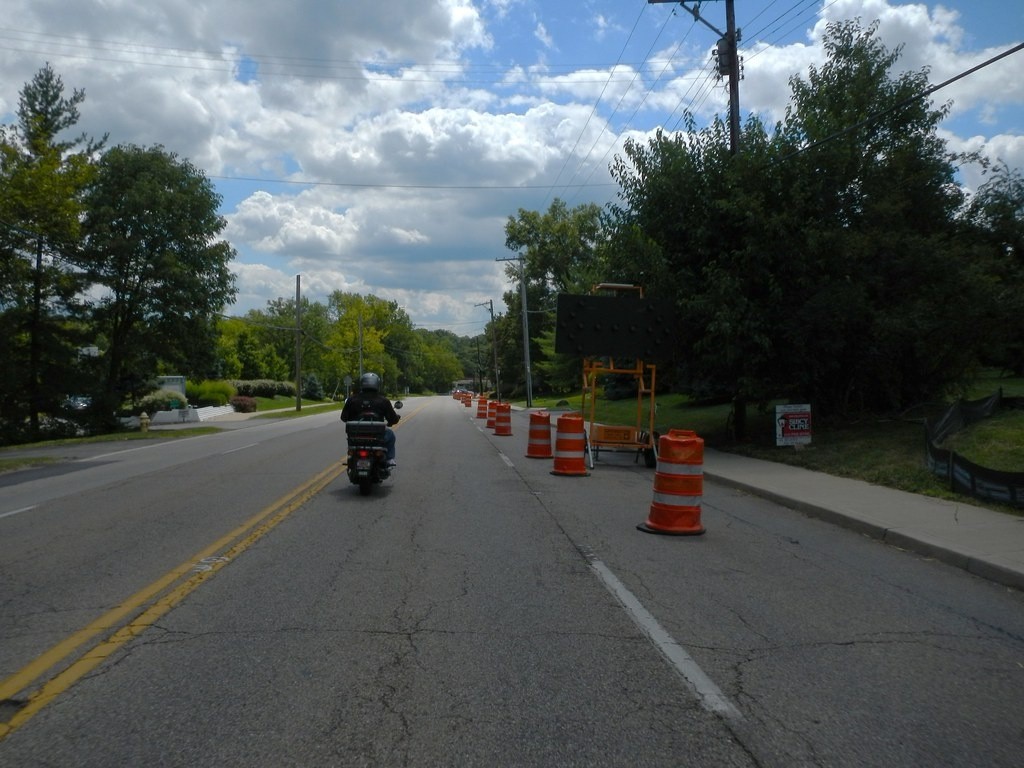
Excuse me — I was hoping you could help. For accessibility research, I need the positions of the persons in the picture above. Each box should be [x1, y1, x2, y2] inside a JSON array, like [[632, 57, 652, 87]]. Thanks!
[[340, 372, 401, 466]]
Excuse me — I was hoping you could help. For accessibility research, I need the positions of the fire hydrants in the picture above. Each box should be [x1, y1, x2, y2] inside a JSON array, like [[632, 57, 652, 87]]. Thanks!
[[139, 412, 150, 433]]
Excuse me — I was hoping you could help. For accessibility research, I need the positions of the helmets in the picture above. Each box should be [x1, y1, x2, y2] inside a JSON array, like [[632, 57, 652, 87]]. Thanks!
[[360, 373, 379, 391]]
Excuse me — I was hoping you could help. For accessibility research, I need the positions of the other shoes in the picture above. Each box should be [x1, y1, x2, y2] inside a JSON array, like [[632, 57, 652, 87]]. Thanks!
[[387, 459, 397, 466]]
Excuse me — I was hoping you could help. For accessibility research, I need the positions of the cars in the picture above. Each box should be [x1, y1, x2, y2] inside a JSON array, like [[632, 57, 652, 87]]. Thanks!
[[60, 398, 91, 409]]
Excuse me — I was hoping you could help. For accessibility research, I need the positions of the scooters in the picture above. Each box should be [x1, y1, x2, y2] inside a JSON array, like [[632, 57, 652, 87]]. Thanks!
[[342, 397, 404, 496]]
